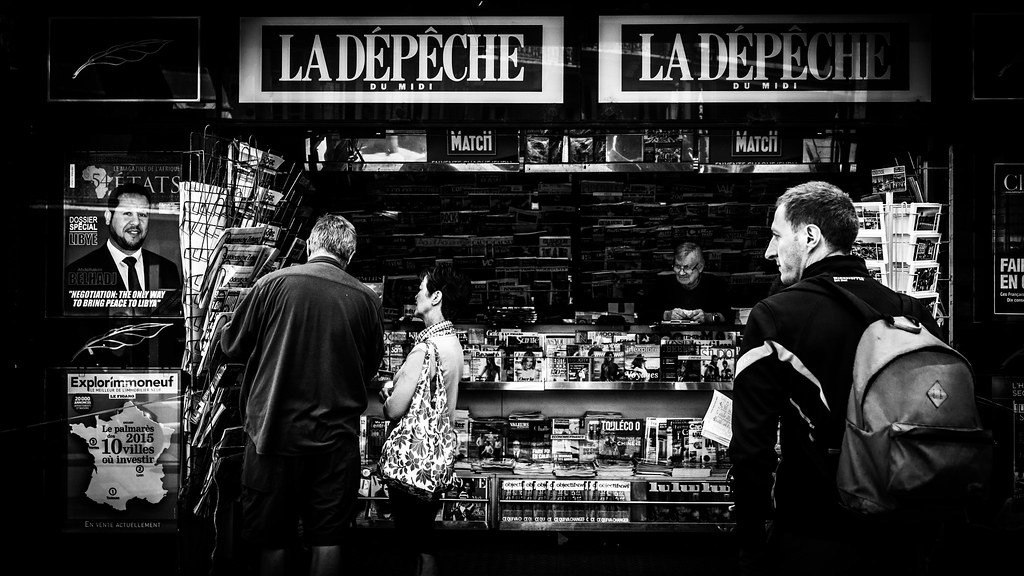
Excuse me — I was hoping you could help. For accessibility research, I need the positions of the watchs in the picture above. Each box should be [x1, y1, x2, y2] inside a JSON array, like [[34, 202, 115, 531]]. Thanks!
[[712, 312, 720, 324]]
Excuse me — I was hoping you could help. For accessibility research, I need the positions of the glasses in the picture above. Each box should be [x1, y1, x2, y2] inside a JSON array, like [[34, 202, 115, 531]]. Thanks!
[[672, 263, 700, 274]]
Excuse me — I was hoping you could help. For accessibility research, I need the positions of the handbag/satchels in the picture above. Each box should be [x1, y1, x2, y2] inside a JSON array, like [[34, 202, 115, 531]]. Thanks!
[[378, 339, 455, 493]]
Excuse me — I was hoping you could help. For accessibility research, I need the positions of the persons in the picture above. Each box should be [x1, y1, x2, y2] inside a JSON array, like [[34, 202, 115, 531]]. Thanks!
[[643, 243, 738, 324], [377, 266, 465, 576], [732, 180, 950, 576], [721, 360, 733, 382], [219, 215, 386, 576], [65, 183, 181, 291], [631, 353, 652, 382], [600, 352, 622, 382], [477, 353, 501, 383], [517, 352, 541, 381], [704, 354, 720, 381]]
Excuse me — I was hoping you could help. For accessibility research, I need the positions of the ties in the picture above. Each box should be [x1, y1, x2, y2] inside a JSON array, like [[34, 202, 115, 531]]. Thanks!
[[122, 257, 143, 295]]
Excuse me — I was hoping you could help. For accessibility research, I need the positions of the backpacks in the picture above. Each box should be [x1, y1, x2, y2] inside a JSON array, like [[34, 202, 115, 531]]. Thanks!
[[775, 273, 988, 529]]
